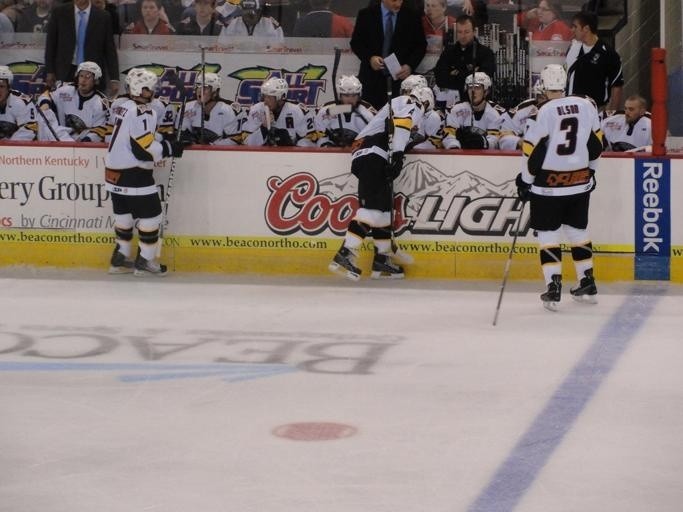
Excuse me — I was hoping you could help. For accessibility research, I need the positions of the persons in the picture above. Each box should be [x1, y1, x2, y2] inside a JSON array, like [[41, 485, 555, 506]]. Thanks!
[[348, 0, 428, 110], [433, 15, 498, 104], [515, 64, 610, 310], [223, 0, 284, 36], [399, 74, 443, 149], [461, 0, 476, 16], [289, 0, 352, 36], [563, 10, 625, 122], [122, 0, 175, 35], [327, 85, 435, 282], [515, 0, 572, 41], [423, 0, 456, 36], [241, 76, 307, 147], [104, 68, 176, 145], [600, 93, 653, 153], [44, 0, 121, 99], [91, 0, 121, 33], [174, 71, 241, 146], [15, 0, 55, 32], [34, 61, 109, 143], [0, 64, 39, 142], [175, 0, 223, 36], [497, 79, 548, 151], [102, 66, 188, 279], [312, 74, 378, 147], [442, 72, 501, 150]]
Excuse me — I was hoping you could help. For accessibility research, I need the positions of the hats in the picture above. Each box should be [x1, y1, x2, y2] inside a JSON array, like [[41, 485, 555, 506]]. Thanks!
[[235, 0, 263, 16]]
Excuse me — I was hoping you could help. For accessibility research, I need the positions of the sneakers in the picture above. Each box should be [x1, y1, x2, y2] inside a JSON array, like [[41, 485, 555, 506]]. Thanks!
[[110, 243, 134, 268], [541, 274, 562, 301], [372, 246, 402, 272], [334, 239, 363, 274], [134, 244, 166, 273], [570, 269, 598, 294]]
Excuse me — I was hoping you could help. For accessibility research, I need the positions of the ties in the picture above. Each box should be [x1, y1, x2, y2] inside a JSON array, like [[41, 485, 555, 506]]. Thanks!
[[383, 12, 393, 59], [75, 11, 87, 65]]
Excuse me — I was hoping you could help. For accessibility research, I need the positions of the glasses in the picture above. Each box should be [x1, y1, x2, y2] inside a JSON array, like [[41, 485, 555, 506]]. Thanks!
[[538, 6, 553, 11]]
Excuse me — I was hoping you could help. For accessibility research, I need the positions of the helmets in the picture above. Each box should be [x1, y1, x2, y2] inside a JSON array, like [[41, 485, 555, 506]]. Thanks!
[[261, 76, 288, 101], [464, 71, 492, 92], [539, 63, 567, 91], [195, 71, 221, 93], [124, 67, 158, 97], [337, 74, 362, 97], [533, 79, 544, 97], [400, 74, 427, 91], [0, 66, 13, 84], [74, 61, 102, 80], [410, 85, 435, 112]]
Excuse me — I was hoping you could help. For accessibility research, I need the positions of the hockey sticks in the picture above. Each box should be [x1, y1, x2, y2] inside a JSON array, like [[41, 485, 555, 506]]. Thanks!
[[387, 91, 415, 265], [332, 49, 346, 147], [156, 76, 189, 258]]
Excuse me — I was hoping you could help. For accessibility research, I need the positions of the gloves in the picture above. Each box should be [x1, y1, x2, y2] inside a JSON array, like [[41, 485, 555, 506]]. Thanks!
[[515, 173, 531, 202], [461, 133, 489, 150], [384, 150, 404, 181], [161, 136, 184, 159], [270, 129, 293, 146]]
